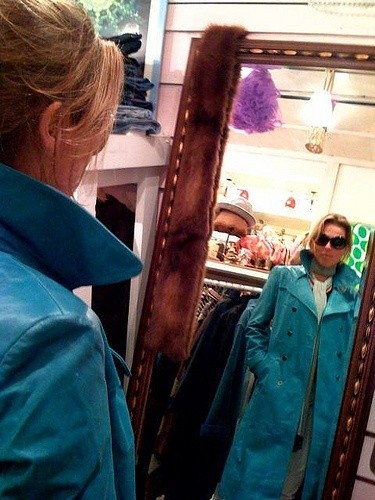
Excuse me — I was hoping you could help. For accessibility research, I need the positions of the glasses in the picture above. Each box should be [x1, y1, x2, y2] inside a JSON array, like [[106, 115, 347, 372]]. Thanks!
[[92, 114, 115, 156], [315, 231, 348, 250]]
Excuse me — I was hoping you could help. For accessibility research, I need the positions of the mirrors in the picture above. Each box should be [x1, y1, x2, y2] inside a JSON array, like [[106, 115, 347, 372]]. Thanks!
[[127, 36, 375, 500]]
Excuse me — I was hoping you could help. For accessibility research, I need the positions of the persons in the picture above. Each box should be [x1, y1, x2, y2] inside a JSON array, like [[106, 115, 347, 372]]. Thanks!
[[0, 0, 144, 500], [216, 215, 359, 500]]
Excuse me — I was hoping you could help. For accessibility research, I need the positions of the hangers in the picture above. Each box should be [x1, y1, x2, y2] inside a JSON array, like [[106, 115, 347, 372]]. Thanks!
[[202, 279, 258, 302]]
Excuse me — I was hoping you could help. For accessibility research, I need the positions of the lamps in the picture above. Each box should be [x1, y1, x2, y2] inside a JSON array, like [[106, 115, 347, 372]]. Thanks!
[[300, 71, 337, 155]]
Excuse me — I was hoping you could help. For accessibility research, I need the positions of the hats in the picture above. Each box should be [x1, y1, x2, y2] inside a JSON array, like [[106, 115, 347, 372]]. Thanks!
[[216, 196, 257, 227]]
